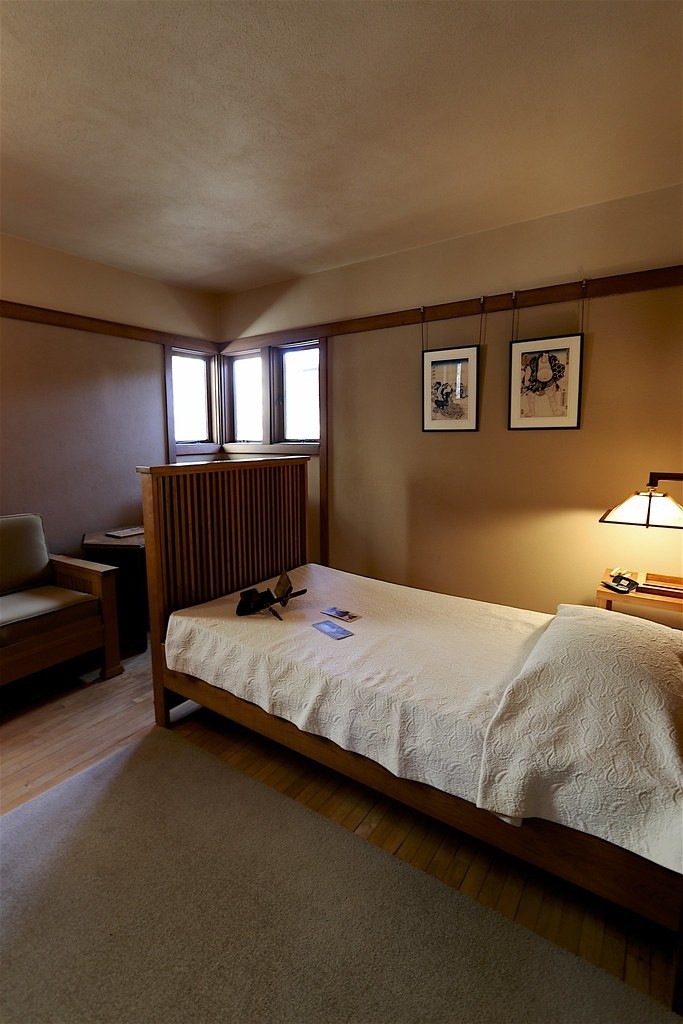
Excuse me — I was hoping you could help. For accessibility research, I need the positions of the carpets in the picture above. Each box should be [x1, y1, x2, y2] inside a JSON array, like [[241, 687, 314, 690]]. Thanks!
[[0, 727, 683, 1024]]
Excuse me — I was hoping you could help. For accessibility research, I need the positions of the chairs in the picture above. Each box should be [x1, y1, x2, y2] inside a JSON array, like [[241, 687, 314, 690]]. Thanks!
[[0, 512, 124, 688]]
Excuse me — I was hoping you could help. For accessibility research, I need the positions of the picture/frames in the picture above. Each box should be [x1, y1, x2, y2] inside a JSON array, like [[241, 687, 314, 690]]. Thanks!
[[421, 345, 480, 432], [508, 333, 584, 430]]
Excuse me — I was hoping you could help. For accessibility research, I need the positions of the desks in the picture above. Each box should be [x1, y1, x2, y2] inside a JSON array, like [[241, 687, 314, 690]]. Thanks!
[[84, 526, 146, 652], [594, 569, 683, 632]]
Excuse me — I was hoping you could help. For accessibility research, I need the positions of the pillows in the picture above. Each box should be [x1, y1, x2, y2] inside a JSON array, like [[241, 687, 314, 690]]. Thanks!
[[473, 604, 683, 873]]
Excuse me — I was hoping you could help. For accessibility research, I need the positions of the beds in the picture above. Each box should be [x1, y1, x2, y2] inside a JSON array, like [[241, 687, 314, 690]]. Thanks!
[[136, 456, 683, 936]]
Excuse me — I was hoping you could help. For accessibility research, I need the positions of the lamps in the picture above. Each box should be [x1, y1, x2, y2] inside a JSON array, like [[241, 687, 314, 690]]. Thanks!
[[599, 472, 683, 529]]
